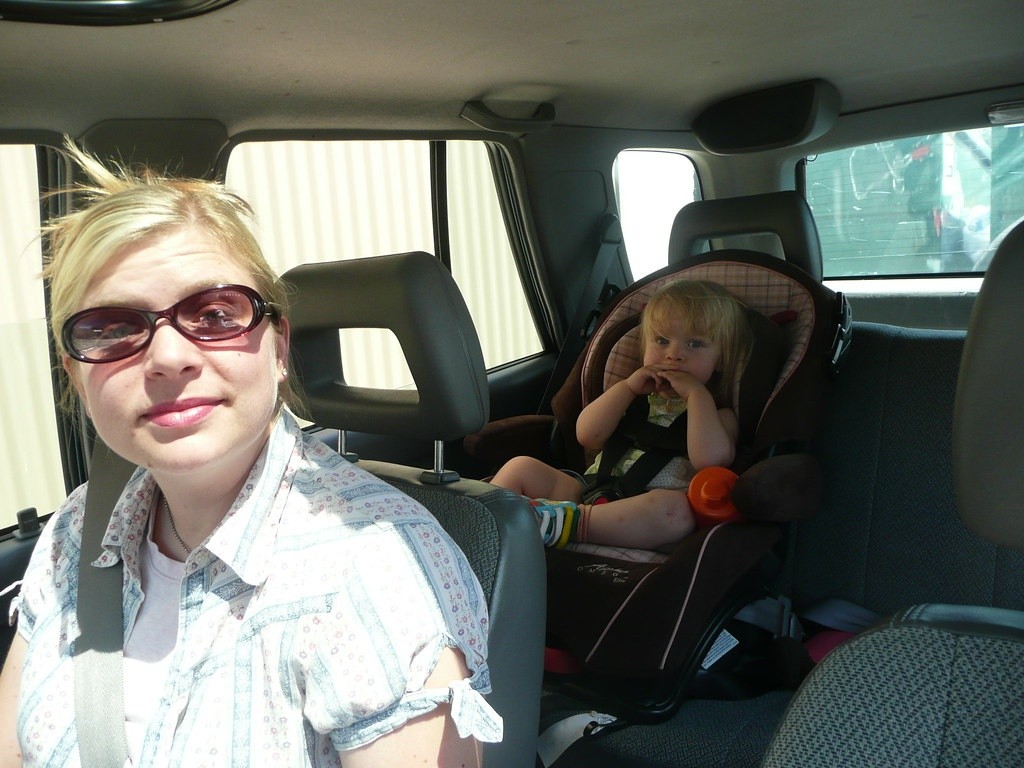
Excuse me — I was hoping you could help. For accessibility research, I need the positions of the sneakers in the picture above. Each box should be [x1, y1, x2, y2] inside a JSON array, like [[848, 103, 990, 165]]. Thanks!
[[530, 497, 578, 547]]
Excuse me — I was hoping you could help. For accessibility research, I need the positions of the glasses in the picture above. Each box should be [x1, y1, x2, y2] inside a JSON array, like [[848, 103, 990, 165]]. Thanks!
[[61, 284, 278, 363]]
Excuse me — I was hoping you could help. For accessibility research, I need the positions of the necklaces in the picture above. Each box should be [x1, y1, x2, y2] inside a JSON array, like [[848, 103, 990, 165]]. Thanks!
[[159, 495, 193, 557]]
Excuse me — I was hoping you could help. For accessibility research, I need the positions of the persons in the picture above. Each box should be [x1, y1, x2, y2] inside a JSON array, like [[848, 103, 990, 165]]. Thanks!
[[482, 278, 753, 550], [0, 134, 502, 768]]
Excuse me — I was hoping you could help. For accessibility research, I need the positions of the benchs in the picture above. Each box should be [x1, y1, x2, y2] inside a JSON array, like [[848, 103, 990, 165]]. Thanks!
[[538, 190, 1024, 768]]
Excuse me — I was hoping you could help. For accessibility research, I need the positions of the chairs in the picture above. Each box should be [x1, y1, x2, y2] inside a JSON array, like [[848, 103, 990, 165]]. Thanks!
[[759, 220, 1024, 768], [272, 252, 548, 768], [461, 248, 852, 726]]
